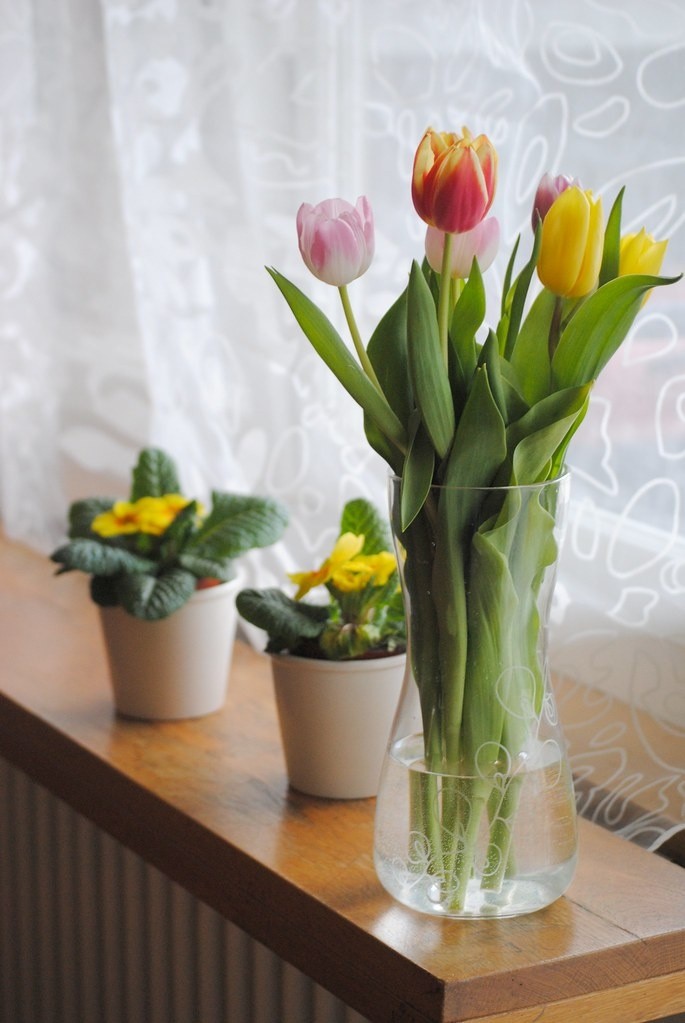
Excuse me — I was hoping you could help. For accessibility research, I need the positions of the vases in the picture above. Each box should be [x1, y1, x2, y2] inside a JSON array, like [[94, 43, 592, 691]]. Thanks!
[[98, 555, 247, 719], [266, 650, 407, 800], [368, 464, 579, 919]]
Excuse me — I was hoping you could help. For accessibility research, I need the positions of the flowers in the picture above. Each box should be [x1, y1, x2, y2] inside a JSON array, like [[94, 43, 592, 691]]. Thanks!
[[48, 448, 289, 622], [255, 125, 684, 919], [235, 497, 407, 660]]
